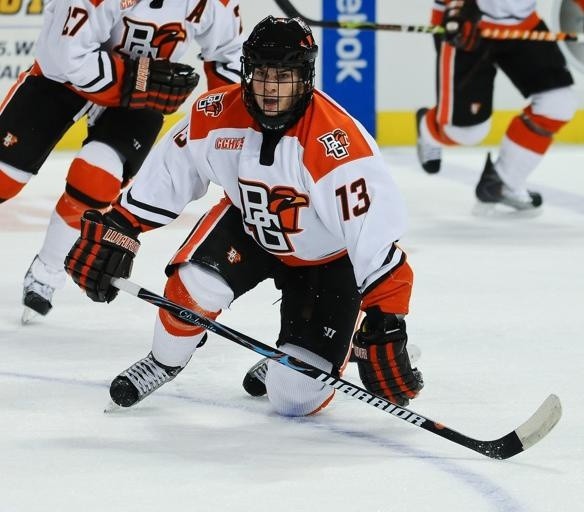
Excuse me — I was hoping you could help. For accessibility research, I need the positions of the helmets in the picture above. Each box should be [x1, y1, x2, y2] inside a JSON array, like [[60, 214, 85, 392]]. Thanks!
[[238, 14, 319, 133]]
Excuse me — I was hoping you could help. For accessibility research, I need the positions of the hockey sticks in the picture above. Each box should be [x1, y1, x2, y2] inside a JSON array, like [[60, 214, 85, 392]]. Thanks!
[[276, 1, 583, 42], [112, 276, 562, 460]]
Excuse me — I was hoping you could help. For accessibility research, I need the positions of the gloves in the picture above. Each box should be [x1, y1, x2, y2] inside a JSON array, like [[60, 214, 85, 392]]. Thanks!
[[440, 0, 484, 51], [352, 304, 425, 407], [121, 54, 201, 118], [64, 206, 141, 304]]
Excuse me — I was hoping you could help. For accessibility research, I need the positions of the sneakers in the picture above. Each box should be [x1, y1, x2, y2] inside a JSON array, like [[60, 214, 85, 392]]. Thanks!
[[242, 357, 269, 397], [415, 107, 443, 174], [109, 349, 193, 408], [475, 151, 543, 211], [22, 254, 56, 317]]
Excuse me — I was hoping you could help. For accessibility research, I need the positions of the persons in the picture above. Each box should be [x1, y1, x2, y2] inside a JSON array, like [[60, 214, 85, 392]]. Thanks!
[[0, 0, 244, 318], [61, 14, 423, 418], [416, 1, 584, 211]]
